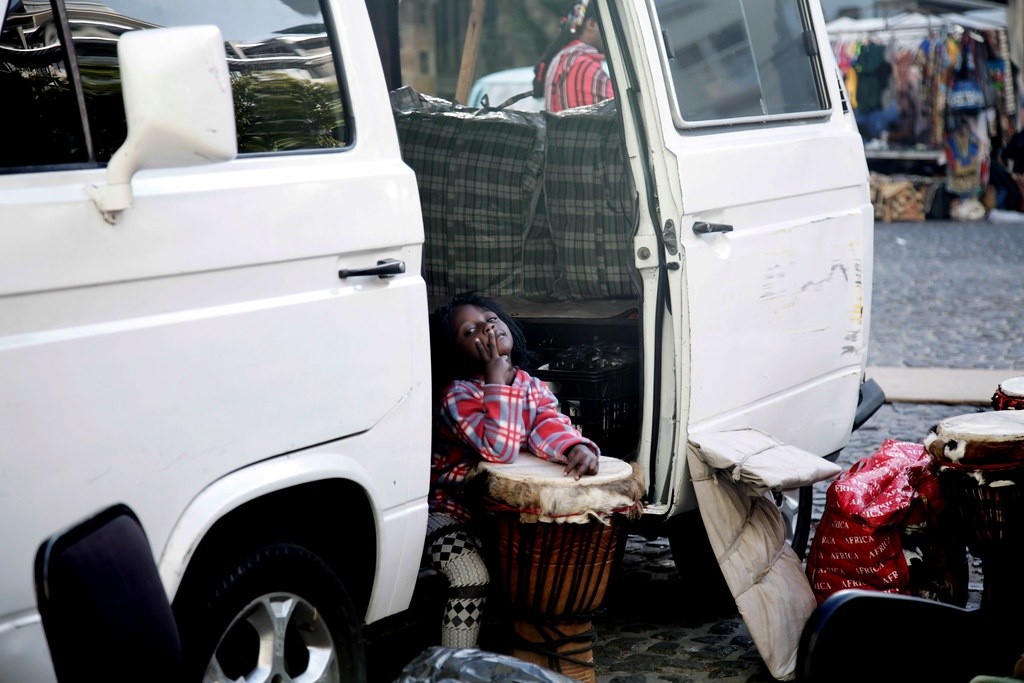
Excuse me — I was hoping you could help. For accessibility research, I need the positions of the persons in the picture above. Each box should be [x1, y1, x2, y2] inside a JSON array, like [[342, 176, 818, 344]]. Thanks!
[[422, 292, 600, 650], [531, 1, 616, 112]]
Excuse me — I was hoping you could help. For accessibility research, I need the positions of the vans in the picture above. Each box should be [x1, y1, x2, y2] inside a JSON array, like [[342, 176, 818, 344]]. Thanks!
[[0, 0, 878, 682]]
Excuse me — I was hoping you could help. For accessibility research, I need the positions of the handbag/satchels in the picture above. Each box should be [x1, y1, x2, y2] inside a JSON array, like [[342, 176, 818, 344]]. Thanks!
[[806, 438, 942, 606]]
